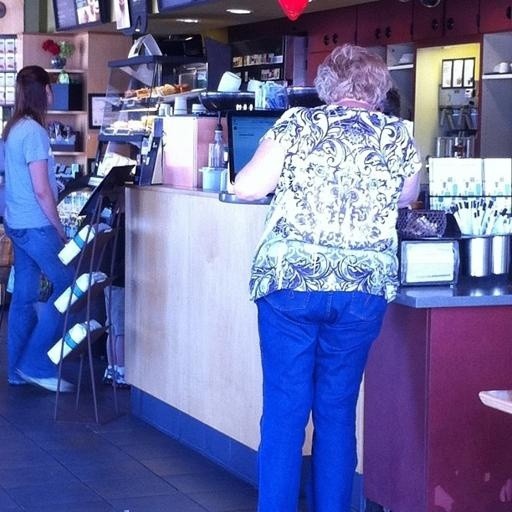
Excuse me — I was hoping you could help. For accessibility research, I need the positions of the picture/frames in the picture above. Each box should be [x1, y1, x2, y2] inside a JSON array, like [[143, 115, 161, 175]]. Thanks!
[[89, 94, 127, 130]]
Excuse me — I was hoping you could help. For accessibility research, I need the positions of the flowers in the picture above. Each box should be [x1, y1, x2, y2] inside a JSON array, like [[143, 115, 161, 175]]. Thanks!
[[41, 39, 76, 56]]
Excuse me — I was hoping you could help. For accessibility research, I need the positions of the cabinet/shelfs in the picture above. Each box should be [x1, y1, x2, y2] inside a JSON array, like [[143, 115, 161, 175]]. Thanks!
[[480, 0, 512, 34], [412, 0, 479, 49], [305, 4, 356, 87], [44, 70, 87, 190], [231, 35, 295, 84], [46, 167, 135, 423], [356, 0, 412, 48]]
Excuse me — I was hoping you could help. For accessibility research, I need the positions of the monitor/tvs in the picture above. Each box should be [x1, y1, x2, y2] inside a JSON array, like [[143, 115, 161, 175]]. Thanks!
[[227, 109, 286, 196], [87, 90, 140, 132]]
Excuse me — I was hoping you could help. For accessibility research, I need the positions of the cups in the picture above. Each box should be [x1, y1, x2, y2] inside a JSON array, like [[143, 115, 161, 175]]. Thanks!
[[489, 233, 511, 274], [173, 96, 188, 114], [200, 167, 227, 193], [247, 79, 267, 94], [458, 234, 490, 279], [217, 70, 242, 92]]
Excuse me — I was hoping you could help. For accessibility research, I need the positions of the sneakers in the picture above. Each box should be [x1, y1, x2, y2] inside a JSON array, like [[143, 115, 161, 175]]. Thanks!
[[9, 369, 72, 393], [103, 368, 129, 388]]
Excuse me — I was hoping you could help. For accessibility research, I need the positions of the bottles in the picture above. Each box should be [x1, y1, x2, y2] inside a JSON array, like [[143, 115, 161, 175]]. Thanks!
[[210, 124, 226, 168]]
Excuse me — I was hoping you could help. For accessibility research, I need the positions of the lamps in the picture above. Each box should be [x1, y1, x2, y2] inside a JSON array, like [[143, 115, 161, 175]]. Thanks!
[[277, 0, 311, 22]]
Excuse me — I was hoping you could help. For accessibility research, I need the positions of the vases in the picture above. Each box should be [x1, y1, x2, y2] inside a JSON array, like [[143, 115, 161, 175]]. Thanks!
[[50, 57, 67, 69]]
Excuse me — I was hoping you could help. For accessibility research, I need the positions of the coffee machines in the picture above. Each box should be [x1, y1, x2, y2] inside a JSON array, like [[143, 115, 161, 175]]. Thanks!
[[434, 84, 476, 157]]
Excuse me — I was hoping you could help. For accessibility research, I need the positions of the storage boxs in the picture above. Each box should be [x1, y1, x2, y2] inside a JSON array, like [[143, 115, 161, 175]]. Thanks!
[[48, 84, 81, 111]]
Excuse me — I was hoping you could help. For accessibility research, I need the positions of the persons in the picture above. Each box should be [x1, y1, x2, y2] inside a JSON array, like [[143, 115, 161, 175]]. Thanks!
[[1, 65, 76, 393], [233, 43, 422, 512]]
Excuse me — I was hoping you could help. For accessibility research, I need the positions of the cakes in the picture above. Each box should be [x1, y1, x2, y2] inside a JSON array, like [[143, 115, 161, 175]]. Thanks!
[[109, 80, 190, 136]]
